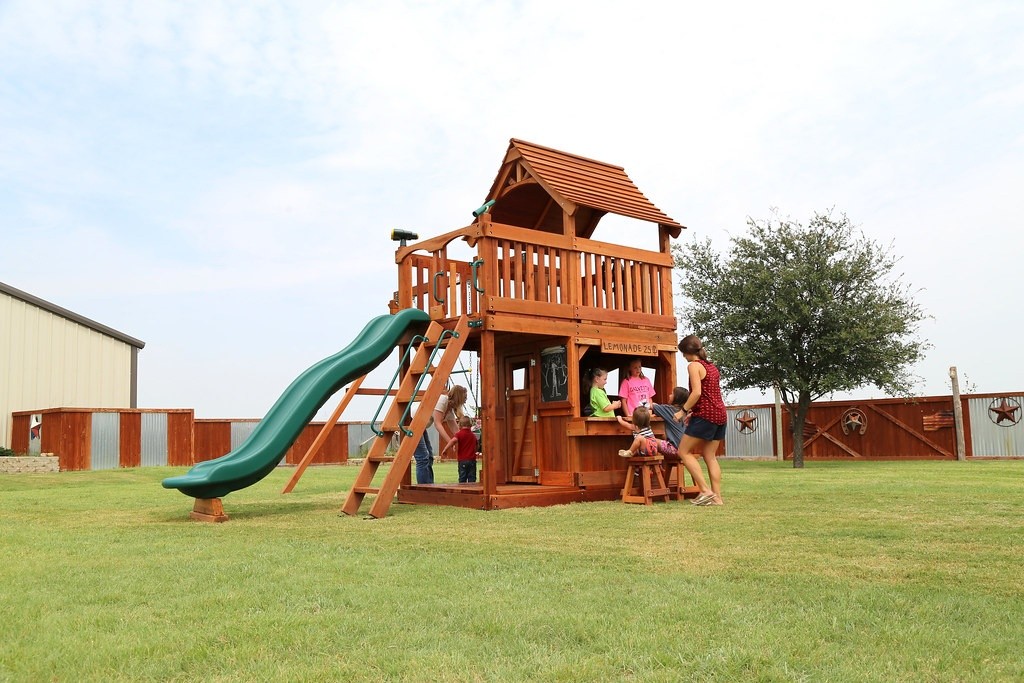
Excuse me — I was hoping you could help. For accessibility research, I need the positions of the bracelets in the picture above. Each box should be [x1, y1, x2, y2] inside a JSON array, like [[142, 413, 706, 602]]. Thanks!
[[681, 406, 688, 415]]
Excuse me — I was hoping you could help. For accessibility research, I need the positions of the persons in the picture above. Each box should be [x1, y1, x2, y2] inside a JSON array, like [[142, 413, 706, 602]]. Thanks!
[[618, 355, 657, 417], [442, 415, 480, 482], [651, 387, 691, 472], [672, 333, 728, 506], [582, 366, 622, 418], [412, 384, 468, 484], [616, 401, 659, 477]]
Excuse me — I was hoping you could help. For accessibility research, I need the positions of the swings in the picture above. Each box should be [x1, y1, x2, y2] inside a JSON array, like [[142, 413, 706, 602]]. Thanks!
[[467, 350, 483, 441]]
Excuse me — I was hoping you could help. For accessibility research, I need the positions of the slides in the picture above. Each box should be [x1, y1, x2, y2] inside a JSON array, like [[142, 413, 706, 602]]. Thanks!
[[161, 308, 431, 499]]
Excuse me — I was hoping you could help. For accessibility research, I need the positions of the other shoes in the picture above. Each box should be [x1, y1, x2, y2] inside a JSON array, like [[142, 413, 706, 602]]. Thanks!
[[689, 493, 716, 504], [660, 468, 666, 472], [634, 471, 640, 476], [650, 470, 653, 475], [619, 450, 634, 457], [698, 499, 723, 505]]
